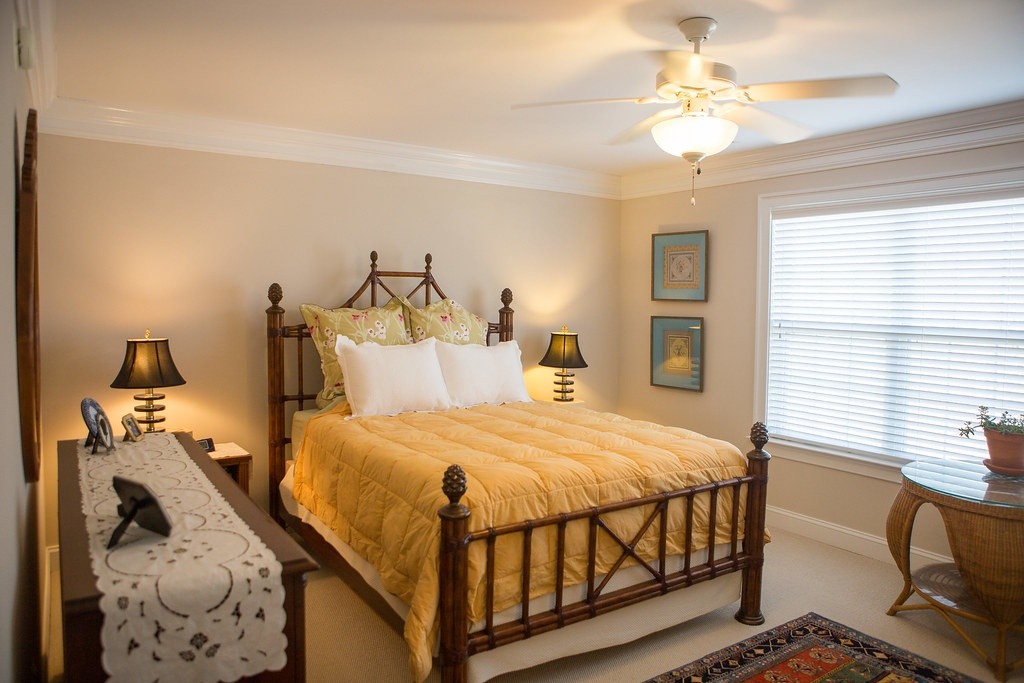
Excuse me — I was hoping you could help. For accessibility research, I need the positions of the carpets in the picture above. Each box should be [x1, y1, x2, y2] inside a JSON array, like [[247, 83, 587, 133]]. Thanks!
[[644, 611, 987, 682]]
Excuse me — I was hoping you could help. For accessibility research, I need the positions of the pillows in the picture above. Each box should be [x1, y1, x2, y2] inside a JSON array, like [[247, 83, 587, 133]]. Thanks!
[[300, 297, 527, 415]]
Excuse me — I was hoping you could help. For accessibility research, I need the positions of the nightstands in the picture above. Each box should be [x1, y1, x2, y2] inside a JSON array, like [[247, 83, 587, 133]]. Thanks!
[[207, 441, 252, 495]]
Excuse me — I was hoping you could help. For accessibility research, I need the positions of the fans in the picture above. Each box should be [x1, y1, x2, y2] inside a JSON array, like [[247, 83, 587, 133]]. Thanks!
[[513, 20, 896, 144]]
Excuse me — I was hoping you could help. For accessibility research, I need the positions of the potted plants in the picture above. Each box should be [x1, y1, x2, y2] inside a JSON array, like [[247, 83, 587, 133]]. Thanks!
[[959, 404, 1024, 476]]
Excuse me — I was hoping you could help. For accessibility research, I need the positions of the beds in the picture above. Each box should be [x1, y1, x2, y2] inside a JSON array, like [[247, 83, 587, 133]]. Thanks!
[[265, 252, 771, 683]]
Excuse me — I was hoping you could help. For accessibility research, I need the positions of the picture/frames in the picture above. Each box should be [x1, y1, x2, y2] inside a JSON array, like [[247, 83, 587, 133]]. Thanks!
[[82, 399, 171, 549], [650, 315, 704, 392], [652, 230, 706, 300]]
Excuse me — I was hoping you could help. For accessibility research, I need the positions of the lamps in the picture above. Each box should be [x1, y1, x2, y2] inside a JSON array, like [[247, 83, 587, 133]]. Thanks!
[[539, 326, 588, 402], [652, 115, 739, 207], [111, 331, 185, 431]]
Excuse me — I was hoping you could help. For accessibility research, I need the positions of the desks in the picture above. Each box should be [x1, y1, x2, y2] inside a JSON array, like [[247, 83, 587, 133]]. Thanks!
[[57, 434, 318, 683], [886, 457, 1024, 683]]
